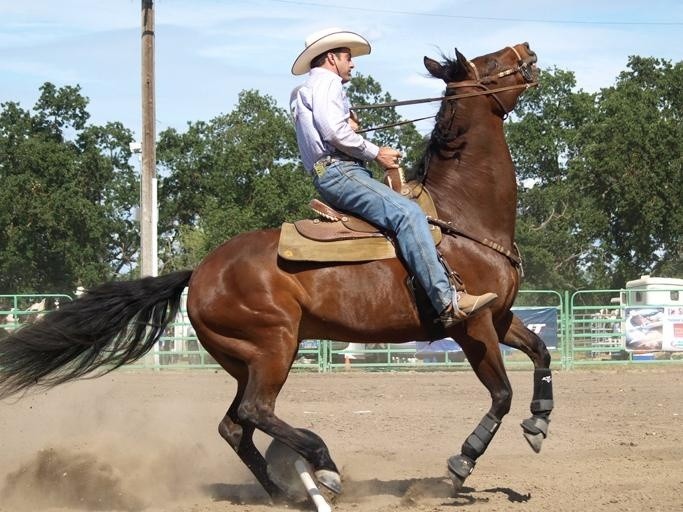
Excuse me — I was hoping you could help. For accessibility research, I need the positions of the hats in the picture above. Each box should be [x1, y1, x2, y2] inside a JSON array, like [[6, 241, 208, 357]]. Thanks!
[[290, 28, 372, 77]]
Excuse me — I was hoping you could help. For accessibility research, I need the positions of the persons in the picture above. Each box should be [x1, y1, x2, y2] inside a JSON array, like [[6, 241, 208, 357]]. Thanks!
[[287, 23, 499, 331]]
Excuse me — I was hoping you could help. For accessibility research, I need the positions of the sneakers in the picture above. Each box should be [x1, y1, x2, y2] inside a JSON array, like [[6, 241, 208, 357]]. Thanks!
[[439, 288, 499, 329]]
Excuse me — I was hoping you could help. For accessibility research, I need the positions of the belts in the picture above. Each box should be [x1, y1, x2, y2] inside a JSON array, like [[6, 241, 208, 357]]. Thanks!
[[310, 152, 358, 178]]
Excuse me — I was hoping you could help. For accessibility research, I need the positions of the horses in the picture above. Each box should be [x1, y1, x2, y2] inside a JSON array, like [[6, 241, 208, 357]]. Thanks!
[[0, 42, 554, 511]]
